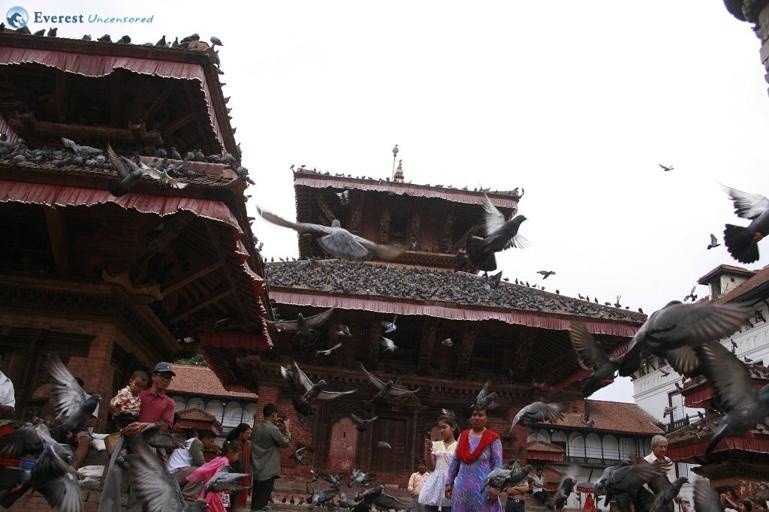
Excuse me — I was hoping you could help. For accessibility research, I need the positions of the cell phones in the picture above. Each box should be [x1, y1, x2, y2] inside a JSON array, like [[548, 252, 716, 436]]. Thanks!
[[426, 432, 431, 441]]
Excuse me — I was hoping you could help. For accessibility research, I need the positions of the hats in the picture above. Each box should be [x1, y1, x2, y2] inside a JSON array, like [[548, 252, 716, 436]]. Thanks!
[[153, 362, 176, 377]]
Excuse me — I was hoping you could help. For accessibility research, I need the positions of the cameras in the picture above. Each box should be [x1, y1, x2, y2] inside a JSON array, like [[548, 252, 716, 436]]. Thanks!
[[276, 416, 285, 423]]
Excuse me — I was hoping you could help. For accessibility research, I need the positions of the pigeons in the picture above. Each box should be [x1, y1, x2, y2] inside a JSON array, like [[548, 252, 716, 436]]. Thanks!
[[0, 134, 769, 512], [218, 78, 237, 134], [0, 16, 222, 59]]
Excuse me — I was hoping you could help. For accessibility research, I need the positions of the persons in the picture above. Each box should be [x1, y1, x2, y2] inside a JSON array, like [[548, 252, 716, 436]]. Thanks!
[[252, 403, 290, 512], [0, 370, 17, 419], [408, 459, 431, 512], [532, 467, 551, 504], [444, 406, 503, 512], [505, 459, 530, 512], [641, 432, 689, 512], [71, 375, 100, 469], [418, 414, 460, 512], [720, 481, 769, 512], [107, 361, 252, 512]]
